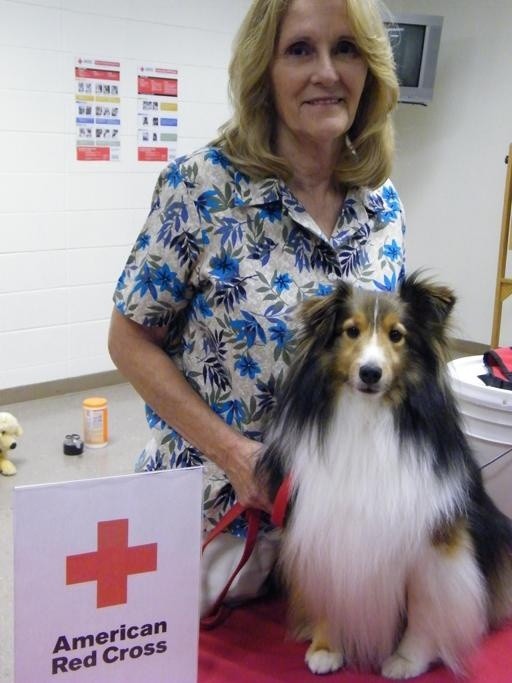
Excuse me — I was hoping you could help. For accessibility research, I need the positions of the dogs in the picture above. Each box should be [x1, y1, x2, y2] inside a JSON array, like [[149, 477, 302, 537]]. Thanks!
[[243, 264, 512, 679]]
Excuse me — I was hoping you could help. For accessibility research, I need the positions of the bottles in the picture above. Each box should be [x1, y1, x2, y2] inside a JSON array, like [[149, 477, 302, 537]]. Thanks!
[[82, 399, 109, 446]]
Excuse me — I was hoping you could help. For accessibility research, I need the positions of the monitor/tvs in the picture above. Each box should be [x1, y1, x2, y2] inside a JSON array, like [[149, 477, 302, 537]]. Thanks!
[[378, 9, 444, 107]]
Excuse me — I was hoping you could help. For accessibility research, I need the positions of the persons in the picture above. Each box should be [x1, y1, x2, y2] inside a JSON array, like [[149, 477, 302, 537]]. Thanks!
[[105, 0, 409, 623]]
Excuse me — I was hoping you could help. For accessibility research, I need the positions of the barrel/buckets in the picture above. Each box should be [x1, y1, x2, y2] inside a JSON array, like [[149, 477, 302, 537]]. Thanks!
[[445, 352, 511, 630]]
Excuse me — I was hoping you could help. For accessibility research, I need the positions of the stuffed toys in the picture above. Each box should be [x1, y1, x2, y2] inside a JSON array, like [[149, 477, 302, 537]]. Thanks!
[[1, 411, 23, 474]]
